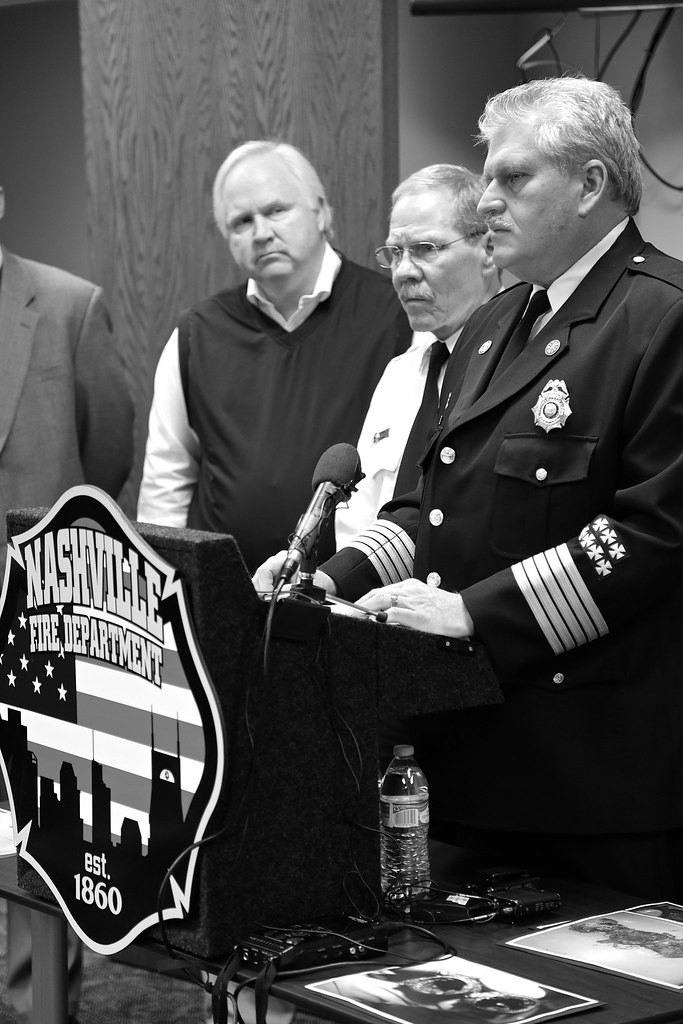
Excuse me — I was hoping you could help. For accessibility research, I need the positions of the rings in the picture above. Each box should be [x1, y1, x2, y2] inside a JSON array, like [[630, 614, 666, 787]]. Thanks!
[[390, 595, 399, 607]]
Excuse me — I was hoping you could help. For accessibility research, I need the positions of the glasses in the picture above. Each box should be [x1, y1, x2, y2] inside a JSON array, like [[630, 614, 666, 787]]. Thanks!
[[373, 230, 489, 268]]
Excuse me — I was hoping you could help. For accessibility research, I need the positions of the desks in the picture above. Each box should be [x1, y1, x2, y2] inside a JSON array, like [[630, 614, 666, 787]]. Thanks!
[[0, 855, 683, 1024]]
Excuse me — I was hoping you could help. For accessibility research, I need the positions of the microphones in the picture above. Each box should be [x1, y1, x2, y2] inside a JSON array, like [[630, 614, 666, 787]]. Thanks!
[[278, 444, 361, 583]]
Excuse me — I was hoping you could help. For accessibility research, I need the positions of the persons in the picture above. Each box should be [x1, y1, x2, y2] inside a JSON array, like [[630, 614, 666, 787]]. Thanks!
[[0, 186, 136, 1022], [131, 78, 682, 1024]]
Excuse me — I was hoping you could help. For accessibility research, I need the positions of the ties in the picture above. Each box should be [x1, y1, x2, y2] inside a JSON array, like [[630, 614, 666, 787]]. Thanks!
[[392, 341, 450, 500], [486, 289, 551, 391]]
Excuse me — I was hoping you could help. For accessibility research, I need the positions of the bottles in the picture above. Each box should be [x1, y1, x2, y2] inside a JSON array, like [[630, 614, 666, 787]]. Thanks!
[[378, 744, 433, 899]]
